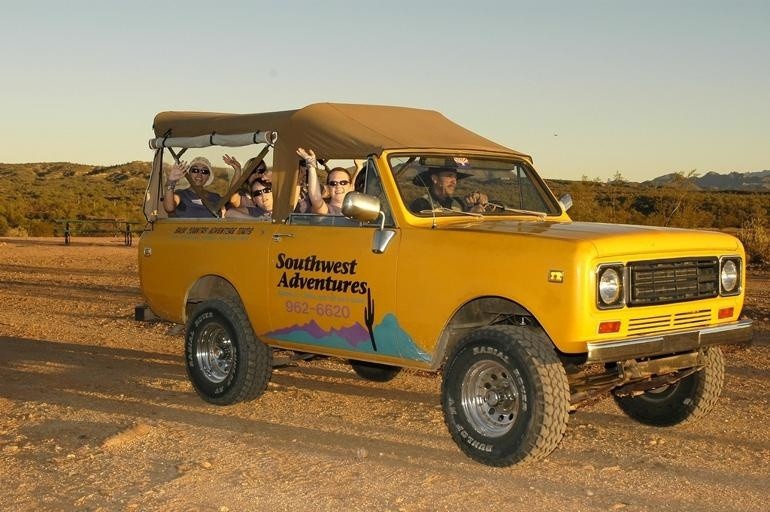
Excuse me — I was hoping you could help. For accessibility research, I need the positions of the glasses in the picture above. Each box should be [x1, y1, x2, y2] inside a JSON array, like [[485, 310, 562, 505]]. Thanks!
[[190, 168, 209, 175], [328, 181, 351, 186], [252, 168, 265, 175], [253, 187, 271, 197]]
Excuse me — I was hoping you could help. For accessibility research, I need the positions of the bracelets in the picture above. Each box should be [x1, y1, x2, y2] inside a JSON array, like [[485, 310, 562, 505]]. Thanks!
[[165, 180, 177, 191]]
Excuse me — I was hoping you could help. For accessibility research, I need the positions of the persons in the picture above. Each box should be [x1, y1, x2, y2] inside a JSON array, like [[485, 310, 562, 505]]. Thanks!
[[408, 154, 498, 216], [164, 156, 224, 219], [219, 146, 365, 223]]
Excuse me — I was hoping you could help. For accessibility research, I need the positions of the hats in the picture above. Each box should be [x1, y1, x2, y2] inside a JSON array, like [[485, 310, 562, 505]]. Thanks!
[[187, 156, 214, 187], [413, 167, 475, 188]]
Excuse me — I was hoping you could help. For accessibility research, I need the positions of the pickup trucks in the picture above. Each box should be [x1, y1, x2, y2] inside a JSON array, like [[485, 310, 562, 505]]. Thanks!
[[135, 101, 757, 475]]
